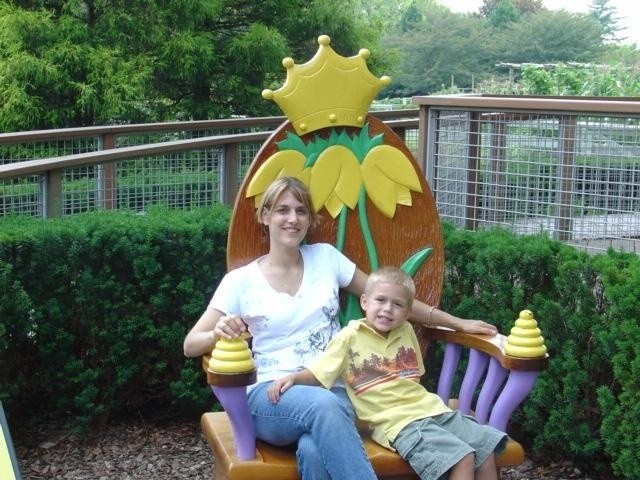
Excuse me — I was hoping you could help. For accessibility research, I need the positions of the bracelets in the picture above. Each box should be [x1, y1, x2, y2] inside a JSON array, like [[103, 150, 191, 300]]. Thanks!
[[427, 305, 436, 326]]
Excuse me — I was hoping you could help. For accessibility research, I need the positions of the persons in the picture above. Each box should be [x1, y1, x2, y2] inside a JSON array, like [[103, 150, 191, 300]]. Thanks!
[[267, 267, 509, 480], [183, 177, 498, 480]]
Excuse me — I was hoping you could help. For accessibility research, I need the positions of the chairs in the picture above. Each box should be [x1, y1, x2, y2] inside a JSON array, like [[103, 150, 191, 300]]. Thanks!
[[201, 34, 550, 480]]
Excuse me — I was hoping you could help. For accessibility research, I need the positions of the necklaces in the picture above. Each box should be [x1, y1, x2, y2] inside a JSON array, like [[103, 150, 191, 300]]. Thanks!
[[266, 257, 302, 296]]
